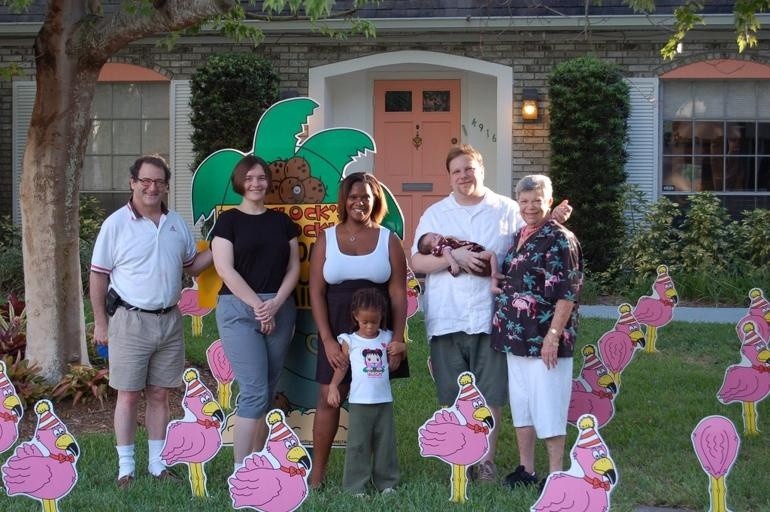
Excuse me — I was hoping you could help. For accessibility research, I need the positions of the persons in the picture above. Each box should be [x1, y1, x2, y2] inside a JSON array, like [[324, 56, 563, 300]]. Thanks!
[[417, 232, 506, 294], [411, 146, 572, 482], [210, 157, 301, 469], [309, 173, 409, 491], [90, 155, 213, 486], [491, 175, 582, 490], [327, 289, 407, 500]]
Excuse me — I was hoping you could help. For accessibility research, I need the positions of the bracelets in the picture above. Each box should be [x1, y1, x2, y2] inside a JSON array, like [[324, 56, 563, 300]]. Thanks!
[[548, 327, 561, 338]]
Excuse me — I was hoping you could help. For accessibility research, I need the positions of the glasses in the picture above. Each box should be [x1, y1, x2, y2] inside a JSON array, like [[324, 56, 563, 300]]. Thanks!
[[135, 177, 165, 188]]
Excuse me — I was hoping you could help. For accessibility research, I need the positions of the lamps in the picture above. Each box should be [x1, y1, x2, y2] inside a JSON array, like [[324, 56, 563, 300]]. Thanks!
[[521, 87, 540, 122]]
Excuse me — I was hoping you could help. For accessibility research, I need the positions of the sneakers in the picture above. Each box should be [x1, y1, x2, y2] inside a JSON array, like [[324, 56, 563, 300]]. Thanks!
[[351, 492, 372, 501], [466, 461, 495, 483], [502, 465, 537, 490], [377, 486, 397, 496], [532, 473, 549, 491]]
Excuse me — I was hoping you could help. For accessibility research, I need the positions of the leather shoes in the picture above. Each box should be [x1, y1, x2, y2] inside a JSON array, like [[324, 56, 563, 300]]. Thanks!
[[117, 473, 136, 490], [152, 466, 185, 484]]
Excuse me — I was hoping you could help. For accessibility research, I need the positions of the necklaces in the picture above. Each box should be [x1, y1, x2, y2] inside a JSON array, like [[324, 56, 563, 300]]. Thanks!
[[521, 224, 543, 239], [341, 223, 370, 242]]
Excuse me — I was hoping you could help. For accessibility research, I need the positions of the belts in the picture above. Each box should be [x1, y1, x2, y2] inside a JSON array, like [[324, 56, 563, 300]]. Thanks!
[[119, 301, 177, 314]]
